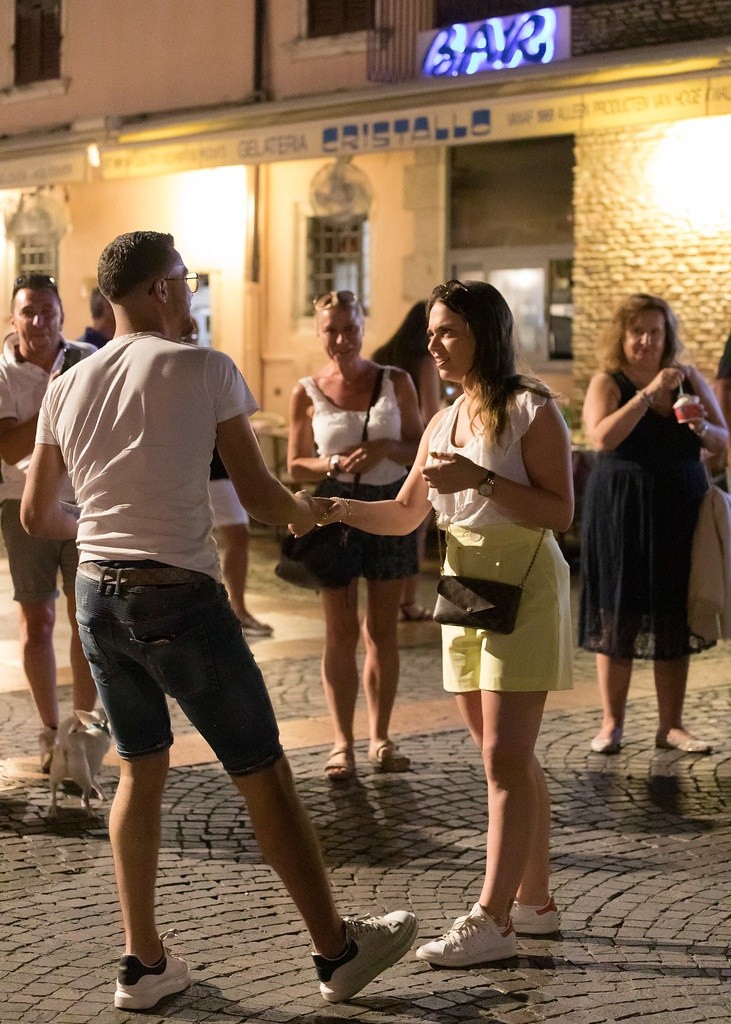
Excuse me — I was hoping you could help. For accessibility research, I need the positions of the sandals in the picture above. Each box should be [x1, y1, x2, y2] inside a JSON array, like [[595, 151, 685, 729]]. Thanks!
[[397, 600, 434, 623], [367, 739, 410, 772], [324, 748, 356, 780]]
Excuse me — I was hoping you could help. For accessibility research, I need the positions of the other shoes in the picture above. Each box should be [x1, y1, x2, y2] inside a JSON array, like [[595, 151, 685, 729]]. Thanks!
[[240, 619, 273, 637], [655, 730, 714, 754], [591, 730, 622, 753], [38, 725, 58, 772]]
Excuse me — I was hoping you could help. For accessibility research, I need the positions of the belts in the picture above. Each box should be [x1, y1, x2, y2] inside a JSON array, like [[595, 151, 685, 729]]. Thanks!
[[77, 561, 210, 588]]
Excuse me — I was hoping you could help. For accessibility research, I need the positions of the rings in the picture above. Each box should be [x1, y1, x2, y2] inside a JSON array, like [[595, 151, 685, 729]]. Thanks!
[[324, 513, 329, 519]]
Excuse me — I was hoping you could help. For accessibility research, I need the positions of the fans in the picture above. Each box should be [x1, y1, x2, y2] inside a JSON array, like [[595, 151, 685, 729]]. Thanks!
[[309, 155, 371, 228]]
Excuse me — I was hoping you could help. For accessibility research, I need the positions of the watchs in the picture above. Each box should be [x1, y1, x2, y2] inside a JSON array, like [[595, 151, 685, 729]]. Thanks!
[[695, 424, 710, 438], [477, 470, 496, 497]]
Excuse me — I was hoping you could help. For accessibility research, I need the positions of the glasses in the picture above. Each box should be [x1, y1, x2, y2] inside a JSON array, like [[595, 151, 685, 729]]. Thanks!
[[13, 274, 56, 288], [432, 279, 473, 301], [312, 289, 357, 311], [147, 272, 200, 296]]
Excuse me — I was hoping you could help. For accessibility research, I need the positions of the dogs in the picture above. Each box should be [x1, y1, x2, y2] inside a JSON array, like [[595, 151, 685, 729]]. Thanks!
[[42, 706, 114, 823]]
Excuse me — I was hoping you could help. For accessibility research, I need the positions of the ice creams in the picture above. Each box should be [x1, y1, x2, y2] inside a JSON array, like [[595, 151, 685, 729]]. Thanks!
[[673, 393, 702, 423]]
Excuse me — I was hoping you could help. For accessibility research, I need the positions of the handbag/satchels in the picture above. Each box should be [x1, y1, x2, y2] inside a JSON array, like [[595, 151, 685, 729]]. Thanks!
[[275, 479, 383, 591], [433, 576, 523, 635]]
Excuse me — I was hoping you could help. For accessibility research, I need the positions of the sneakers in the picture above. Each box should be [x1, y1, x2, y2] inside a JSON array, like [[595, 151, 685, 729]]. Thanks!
[[452, 894, 559, 934], [311, 910, 419, 1003], [416, 902, 518, 966], [114, 928, 191, 1010]]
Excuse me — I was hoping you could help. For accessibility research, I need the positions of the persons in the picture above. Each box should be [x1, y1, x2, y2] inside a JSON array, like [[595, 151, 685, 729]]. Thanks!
[[20, 230, 420, 1012], [75, 285, 116, 350], [715, 332, 731, 492], [209, 446, 276, 636], [0, 274, 98, 775], [576, 292, 730, 753], [367, 296, 451, 622], [287, 289, 425, 781], [313, 277, 573, 969]]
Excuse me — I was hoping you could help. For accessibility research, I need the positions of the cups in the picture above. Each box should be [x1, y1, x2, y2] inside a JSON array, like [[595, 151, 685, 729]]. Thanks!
[[672, 402, 701, 424]]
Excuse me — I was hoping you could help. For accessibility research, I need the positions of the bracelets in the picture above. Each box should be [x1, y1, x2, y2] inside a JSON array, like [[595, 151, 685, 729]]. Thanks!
[[326, 496, 352, 522], [332, 454, 343, 474], [636, 390, 654, 409], [327, 455, 334, 478]]
